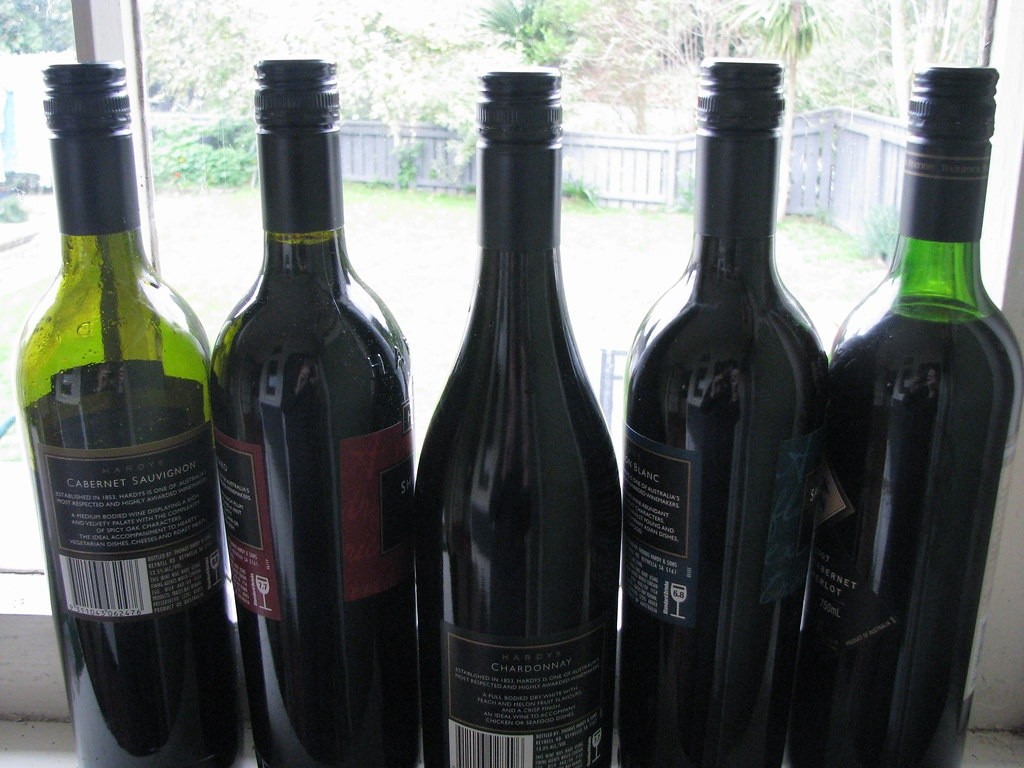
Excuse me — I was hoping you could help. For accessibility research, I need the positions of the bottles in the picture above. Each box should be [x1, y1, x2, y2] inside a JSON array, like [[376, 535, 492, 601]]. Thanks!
[[18, 62, 245, 768], [784, 67, 1023, 768], [624, 62, 832, 768], [212, 59, 418, 768], [417, 69, 624, 768]]
